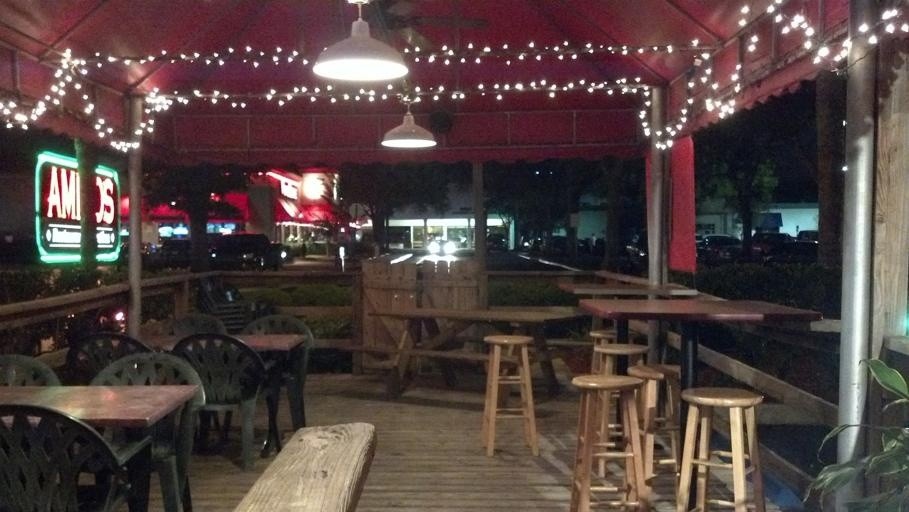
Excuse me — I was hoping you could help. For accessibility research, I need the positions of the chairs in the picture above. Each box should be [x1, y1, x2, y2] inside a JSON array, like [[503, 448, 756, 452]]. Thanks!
[[0, 274, 313, 512]]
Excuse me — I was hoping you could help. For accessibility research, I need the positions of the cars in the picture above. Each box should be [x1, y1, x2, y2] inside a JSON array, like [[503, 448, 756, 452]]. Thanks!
[[0, 233, 291, 349], [428, 239, 455, 256], [523, 230, 817, 268], [486, 233, 510, 253]]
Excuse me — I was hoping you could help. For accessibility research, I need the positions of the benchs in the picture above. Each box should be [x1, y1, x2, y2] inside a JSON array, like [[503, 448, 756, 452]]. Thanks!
[[234, 420, 387, 512], [365, 302, 594, 399]]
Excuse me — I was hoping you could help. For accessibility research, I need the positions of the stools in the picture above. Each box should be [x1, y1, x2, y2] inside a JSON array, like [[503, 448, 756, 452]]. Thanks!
[[587, 326, 684, 485], [677, 386, 770, 510], [480, 333, 544, 460], [568, 374, 645, 512]]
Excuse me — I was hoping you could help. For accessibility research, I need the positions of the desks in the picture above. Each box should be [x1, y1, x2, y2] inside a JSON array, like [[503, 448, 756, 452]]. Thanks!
[[562, 281, 822, 467]]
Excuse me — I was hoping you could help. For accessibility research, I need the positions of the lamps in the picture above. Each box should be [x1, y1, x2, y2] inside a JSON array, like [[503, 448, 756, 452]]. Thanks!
[[379, 99, 437, 150], [309, 1, 410, 84]]
